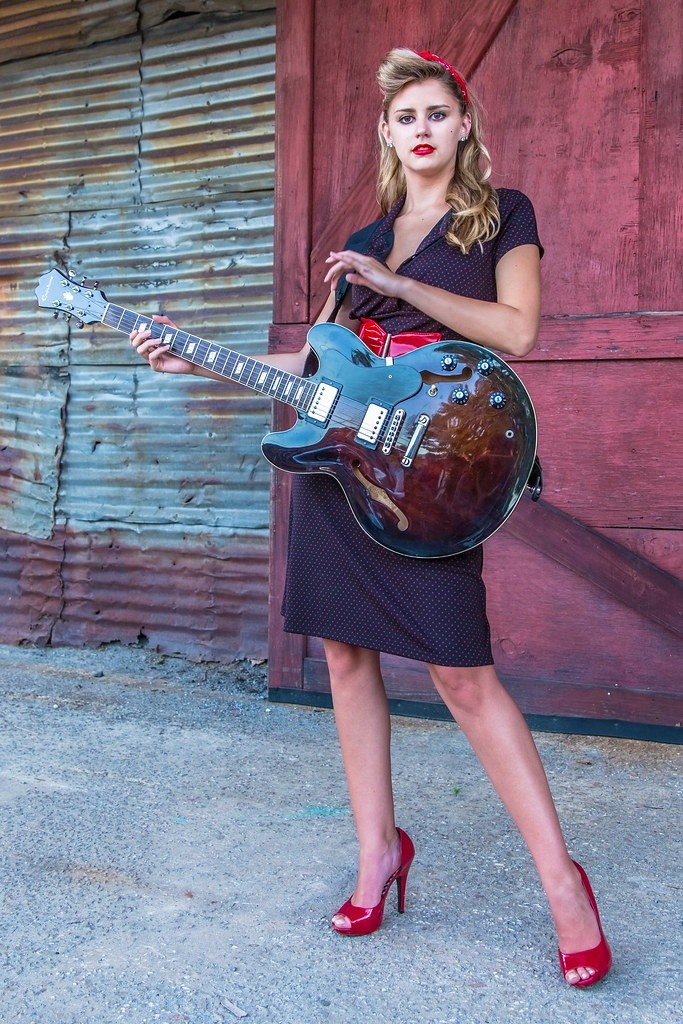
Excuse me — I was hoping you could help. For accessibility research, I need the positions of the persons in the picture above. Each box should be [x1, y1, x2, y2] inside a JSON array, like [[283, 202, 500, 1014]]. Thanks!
[[129, 47, 613, 990]]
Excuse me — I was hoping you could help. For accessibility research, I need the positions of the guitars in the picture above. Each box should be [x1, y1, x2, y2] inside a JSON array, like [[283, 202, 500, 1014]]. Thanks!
[[36, 268, 542, 560]]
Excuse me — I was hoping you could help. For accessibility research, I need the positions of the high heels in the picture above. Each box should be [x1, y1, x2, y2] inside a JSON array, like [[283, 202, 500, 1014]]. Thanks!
[[331, 827, 415, 936], [557, 861, 613, 988]]
